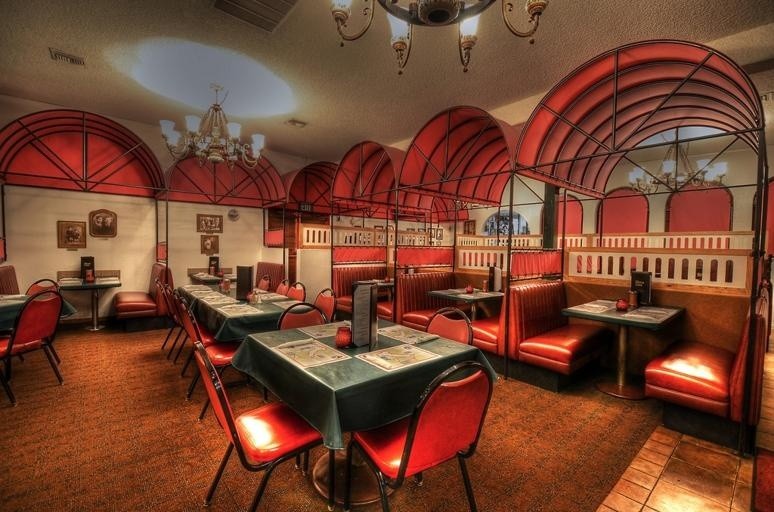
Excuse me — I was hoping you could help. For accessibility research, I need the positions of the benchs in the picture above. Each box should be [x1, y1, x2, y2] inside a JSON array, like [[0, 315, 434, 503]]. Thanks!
[[0, 264, 21, 295], [645, 287, 769, 457], [375, 298, 395, 323], [113, 264, 167, 328], [332, 265, 388, 319], [167, 266, 174, 293], [396, 272, 468, 341], [472, 271, 507, 381], [256, 261, 285, 293], [506, 274, 611, 395]]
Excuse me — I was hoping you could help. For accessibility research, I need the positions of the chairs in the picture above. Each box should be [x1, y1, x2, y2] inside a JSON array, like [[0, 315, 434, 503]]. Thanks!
[[275, 304, 327, 330], [163, 285, 202, 363], [193, 340, 325, 512], [277, 280, 289, 295], [5, 290, 64, 384], [343, 361, 493, 511], [181, 307, 265, 421], [313, 290, 337, 323], [256, 276, 270, 290], [423, 307, 473, 346], [14, 279, 63, 366], [285, 283, 303, 304], [175, 296, 227, 380]]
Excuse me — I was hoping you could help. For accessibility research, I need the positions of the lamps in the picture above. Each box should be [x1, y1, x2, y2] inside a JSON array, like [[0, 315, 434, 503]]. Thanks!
[[160, 82, 267, 170], [627, 140, 727, 195], [325, 0, 563, 75]]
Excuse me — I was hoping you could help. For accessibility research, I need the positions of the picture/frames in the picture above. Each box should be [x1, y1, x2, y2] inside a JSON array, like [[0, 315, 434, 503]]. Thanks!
[[89, 209, 117, 239], [57, 221, 87, 251], [200, 235, 220, 256], [196, 213, 223, 233]]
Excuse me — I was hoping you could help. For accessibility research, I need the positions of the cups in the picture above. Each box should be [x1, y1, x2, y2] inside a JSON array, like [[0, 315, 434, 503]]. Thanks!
[[246, 291, 259, 306], [334, 327, 351, 350], [464, 285, 472, 295], [85, 270, 94, 283], [216, 272, 224, 278], [616, 299, 629, 312]]
